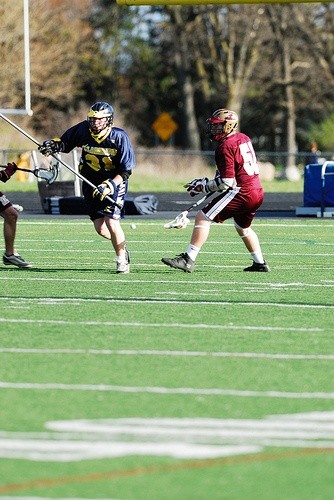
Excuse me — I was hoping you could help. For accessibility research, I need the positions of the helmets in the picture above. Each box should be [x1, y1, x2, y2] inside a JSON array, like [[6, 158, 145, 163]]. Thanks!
[[205, 109, 240, 145], [87, 101, 113, 144]]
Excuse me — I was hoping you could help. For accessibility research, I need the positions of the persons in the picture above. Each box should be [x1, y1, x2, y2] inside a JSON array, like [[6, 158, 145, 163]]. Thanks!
[[37, 102, 131, 274], [0, 162, 32, 268], [304, 144, 319, 165], [15, 153, 28, 181], [161, 110, 270, 273]]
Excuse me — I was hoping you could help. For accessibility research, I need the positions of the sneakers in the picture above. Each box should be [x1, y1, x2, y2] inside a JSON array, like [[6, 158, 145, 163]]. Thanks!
[[162, 253, 194, 274], [3, 250, 29, 268], [116, 247, 130, 273]]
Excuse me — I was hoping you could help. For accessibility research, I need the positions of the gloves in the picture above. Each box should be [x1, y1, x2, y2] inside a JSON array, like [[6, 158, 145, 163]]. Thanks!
[[184, 177, 211, 198], [0, 161, 17, 183], [243, 259, 268, 272], [38, 137, 65, 158], [92, 179, 116, 201]]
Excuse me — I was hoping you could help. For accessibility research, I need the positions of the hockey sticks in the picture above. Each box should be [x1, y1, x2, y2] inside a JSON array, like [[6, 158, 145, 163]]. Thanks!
[[165, 183, 221, 230], [1, 162, 63, 188], [0, 112, 124, 212]]
